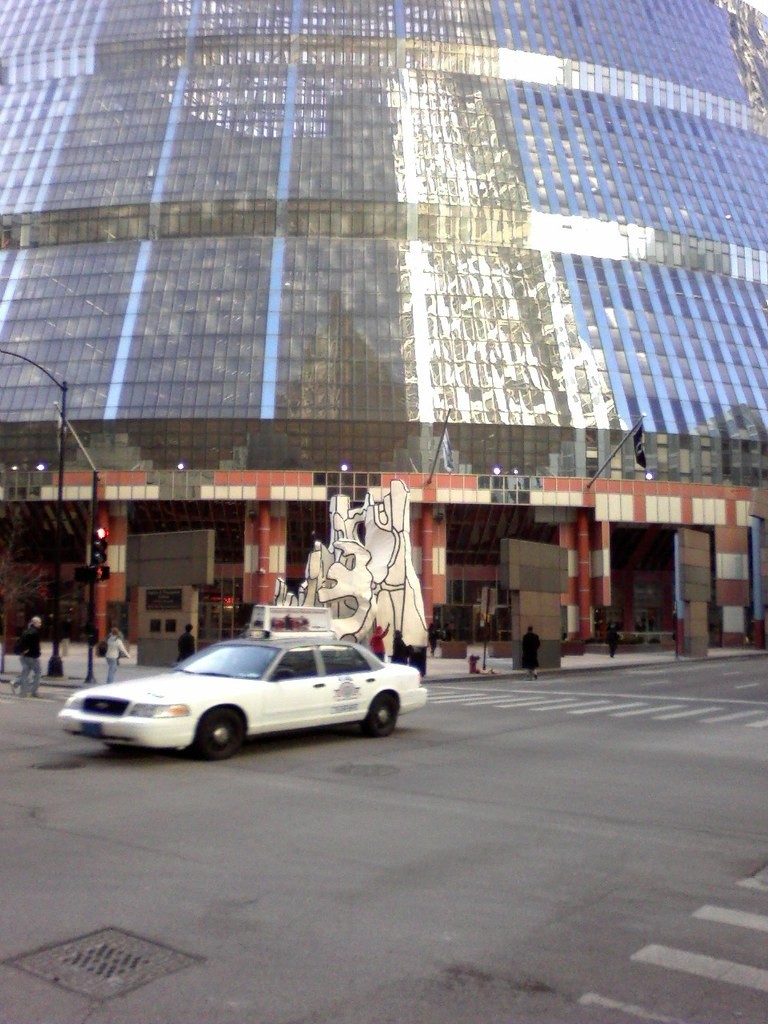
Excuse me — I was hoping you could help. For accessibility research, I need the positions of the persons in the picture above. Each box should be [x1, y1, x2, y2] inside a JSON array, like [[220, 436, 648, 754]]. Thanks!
[[607, 624, 619, 657], [370, 623, 390, 661], [392, 630, 414, 666], [428, 624, 438, 654], [521, 626, 540, 680], [10, 616, 42, 698], [106, 627, 130, 683], [177, 624, 196, 661]]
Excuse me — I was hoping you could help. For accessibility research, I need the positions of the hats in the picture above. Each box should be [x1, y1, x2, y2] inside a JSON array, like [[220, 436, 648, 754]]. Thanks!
[[376, 626, 381, 631], [112, 628, 119, 635]]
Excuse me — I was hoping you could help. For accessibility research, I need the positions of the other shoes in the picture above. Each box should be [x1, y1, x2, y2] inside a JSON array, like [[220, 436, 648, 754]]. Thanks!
[[10, 681, 17, 695]]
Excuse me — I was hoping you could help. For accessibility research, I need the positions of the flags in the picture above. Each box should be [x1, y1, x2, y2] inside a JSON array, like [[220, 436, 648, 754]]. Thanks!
[[633, 424, 646, 469]]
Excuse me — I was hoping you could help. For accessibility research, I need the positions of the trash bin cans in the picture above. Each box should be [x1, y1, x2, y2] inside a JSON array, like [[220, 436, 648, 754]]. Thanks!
[[409, 645, 427, 677]]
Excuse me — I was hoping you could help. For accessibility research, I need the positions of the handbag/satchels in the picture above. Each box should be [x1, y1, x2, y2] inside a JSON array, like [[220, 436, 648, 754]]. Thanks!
[[96, 641, 108, 657]]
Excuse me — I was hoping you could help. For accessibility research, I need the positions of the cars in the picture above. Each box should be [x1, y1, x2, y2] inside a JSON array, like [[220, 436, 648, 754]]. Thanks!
[[56, 605, 429, 762]]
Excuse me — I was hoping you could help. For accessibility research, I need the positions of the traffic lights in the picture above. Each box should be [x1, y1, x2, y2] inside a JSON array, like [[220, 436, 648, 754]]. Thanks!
[[92, 526, 108, 564], [89, 565, 111, 582]]
